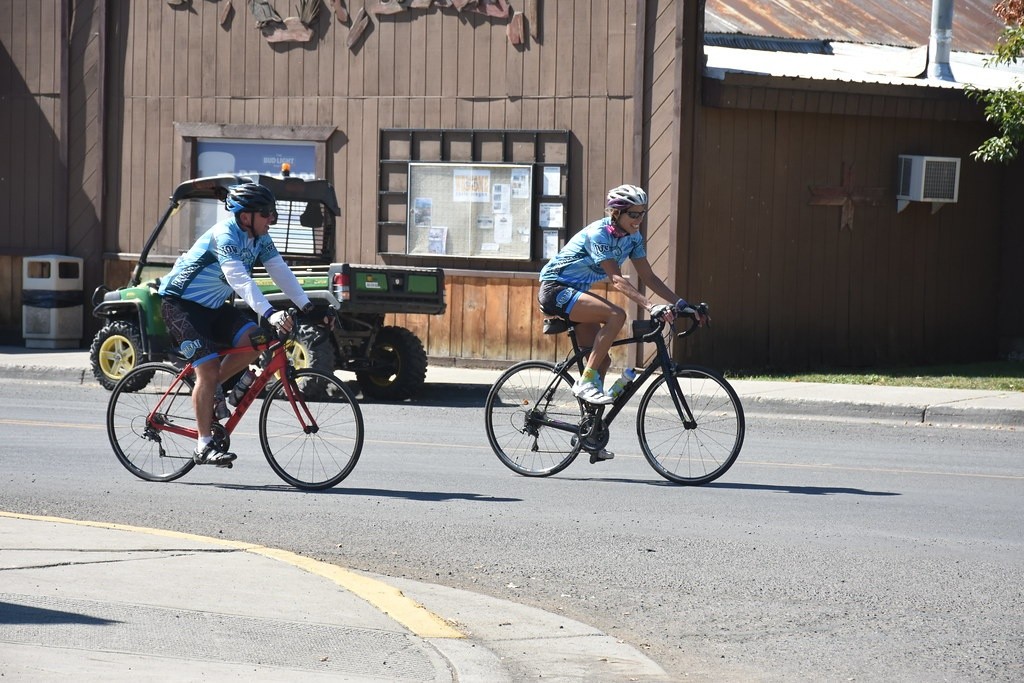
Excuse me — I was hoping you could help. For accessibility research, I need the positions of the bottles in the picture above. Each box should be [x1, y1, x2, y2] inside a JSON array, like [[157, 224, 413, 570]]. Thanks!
[[212, 385, 231, 420], [608, 368, 636, 398], [228, 368, 256, 407], [592, 371, 602, 393]]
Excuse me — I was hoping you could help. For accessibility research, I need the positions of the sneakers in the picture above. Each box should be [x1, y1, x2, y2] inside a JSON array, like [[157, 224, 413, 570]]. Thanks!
[[571, 380, 614, 403], [213, 393, 227, 418], [571, 433, 614, 458], [193, 441, 237, 465]]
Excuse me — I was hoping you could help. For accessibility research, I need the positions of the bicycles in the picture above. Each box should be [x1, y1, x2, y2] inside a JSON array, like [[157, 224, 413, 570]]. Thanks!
[[107, 303, 365, 491], [484, 301, 745, 487]]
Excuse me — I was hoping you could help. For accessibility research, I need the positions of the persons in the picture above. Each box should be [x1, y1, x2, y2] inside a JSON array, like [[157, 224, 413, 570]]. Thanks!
[[539, 184, 712, 459], [158, 184, 337, 465]]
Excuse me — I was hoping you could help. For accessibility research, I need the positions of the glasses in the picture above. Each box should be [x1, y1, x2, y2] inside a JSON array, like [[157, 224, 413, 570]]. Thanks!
[[623, 211, 646, 219], [260, 211, 273, 218]]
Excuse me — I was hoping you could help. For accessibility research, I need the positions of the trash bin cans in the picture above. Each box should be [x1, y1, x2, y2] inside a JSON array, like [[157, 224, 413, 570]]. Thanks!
[[21, 254, 84, 350]]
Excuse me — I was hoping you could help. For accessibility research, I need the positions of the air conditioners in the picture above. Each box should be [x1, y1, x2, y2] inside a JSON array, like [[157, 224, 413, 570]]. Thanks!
[[898, 154, 961, 202]]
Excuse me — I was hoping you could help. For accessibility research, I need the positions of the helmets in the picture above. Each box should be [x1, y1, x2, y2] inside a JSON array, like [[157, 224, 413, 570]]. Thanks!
[[607, 184, 647, 209], [226, 183, 274, 211]]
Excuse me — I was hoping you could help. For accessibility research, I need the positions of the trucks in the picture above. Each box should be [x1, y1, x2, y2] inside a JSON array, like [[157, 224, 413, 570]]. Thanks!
[[89, 163, 447, 403]]
